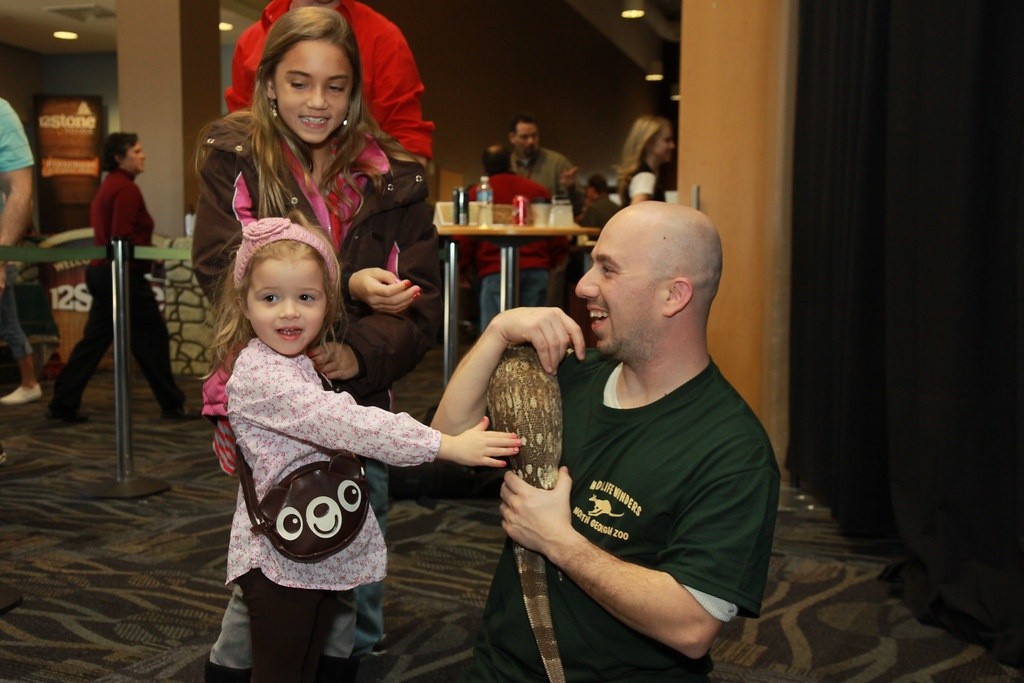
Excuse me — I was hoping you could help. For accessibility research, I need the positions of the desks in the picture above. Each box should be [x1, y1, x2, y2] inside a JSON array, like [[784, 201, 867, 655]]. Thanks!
[[434, 199, 600, 390]]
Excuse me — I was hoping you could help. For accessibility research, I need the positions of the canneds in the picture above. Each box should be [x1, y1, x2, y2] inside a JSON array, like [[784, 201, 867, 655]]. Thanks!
[[515, 194, 529, 226], [452, 185, 470, 225]]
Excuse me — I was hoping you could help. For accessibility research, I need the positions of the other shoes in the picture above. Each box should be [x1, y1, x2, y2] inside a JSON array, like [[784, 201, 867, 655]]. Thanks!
[[47, 413, 91, 426], [0, 384, 43, 405], [159, 407, 203, 421]]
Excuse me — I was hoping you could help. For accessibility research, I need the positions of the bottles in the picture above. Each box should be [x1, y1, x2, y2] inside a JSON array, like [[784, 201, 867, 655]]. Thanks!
[[477, 176, 493, 227], [185, 204, 196, 237]]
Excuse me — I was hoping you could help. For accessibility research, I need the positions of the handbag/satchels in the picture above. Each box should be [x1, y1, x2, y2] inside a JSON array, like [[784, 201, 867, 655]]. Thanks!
[[235, 447, 372, 564]]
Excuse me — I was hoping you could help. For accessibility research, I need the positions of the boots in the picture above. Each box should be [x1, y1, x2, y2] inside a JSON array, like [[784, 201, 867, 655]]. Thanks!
[[204, 659, 253, 683], [314, 655, 360, 683]]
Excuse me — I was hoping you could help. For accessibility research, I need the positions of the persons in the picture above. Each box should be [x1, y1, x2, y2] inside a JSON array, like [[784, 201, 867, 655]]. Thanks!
[[428, 200, 782, 683], [0, 96, 43, 405], [189, 6, 445, 683], [507, 116, 576, 209], [224, 0, 435, 171], [618, 115, 674, 207], [45, 131, 202, 419], [581, 174, 621, 273], [205, 213, 522, 683], [453, 145, 555, 336]]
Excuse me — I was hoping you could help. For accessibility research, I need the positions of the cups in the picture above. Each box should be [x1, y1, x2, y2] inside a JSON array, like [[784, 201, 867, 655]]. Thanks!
[[548, 194, 574, 228], [531, 197, 551, 225]]
